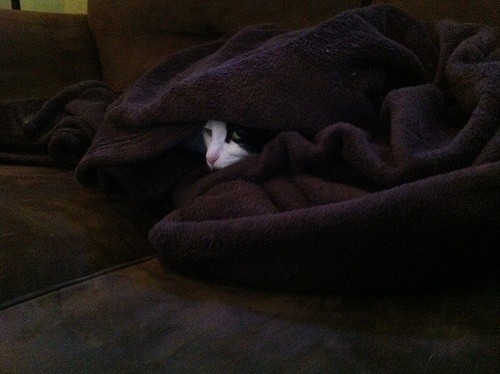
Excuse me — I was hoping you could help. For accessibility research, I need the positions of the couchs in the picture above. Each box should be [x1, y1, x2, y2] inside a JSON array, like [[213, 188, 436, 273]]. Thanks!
[[1, 0, 500, 371]]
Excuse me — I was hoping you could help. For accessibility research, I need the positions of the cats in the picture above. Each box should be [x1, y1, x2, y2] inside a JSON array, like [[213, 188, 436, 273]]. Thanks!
[[202, 120, 274, 171]]
